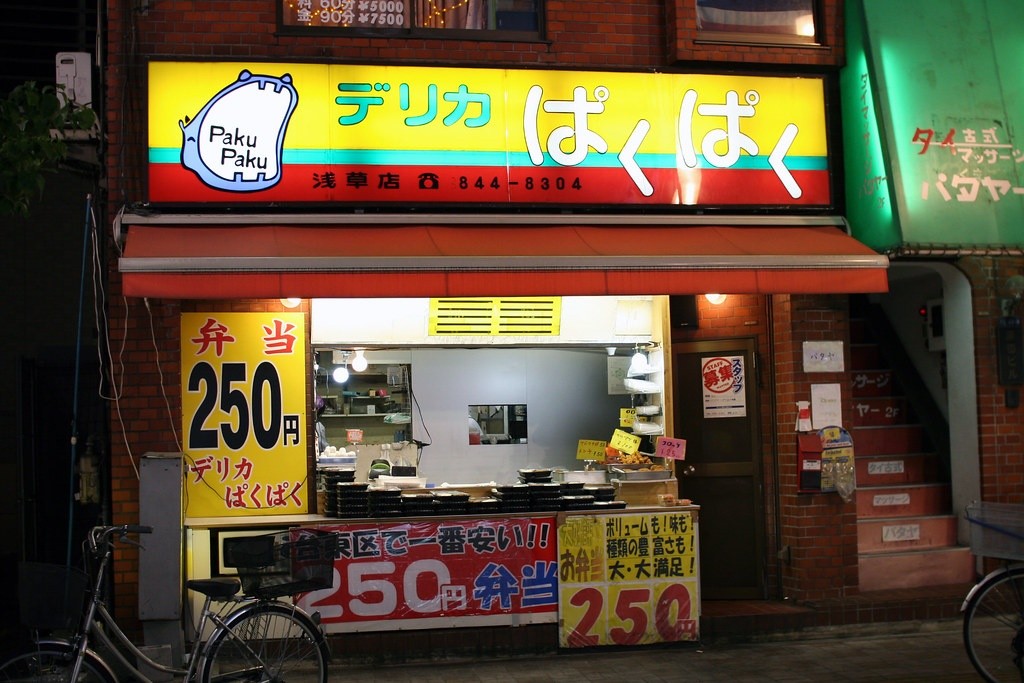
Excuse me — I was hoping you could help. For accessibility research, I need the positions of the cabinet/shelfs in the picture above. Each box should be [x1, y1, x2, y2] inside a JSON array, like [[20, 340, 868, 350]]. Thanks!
[[317, 373, 404, 417]]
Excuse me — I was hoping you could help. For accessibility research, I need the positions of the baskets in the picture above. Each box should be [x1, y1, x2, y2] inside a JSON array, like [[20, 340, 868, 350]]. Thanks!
[[965, 500, 1024, 560], [230, 527, 339, 596]]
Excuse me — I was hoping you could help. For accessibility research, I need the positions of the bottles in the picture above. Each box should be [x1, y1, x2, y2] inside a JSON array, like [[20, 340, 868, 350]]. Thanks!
[[337, 391, 344, 414], [394, 430, 404, 442]]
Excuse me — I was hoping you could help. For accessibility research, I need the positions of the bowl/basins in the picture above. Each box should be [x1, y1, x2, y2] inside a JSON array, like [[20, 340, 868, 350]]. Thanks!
[[324, 468, 470, 507], [498, 469, 616, 504]]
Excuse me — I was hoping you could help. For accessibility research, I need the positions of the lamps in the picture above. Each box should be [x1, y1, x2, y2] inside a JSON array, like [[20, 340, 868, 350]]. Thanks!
[[352, 347, 368, 372], [333, 350, 352, 383]]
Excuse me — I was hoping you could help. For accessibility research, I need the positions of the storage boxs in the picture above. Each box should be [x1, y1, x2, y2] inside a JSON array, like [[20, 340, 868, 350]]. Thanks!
[[367, 405, 375, 414], [323, 467, 628, 517], [368, 388, 376, 396]]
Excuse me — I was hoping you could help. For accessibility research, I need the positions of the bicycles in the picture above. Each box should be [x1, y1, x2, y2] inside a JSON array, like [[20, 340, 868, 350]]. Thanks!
[[1, 524, 333, 683], [959, 499, 1024, 683]]
